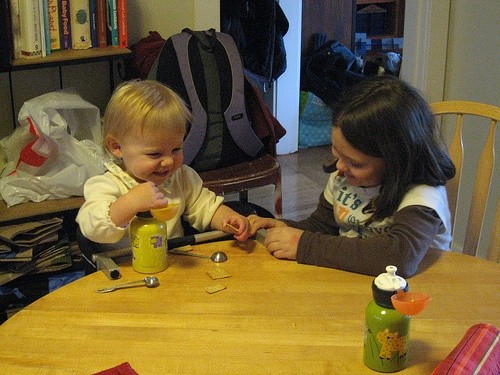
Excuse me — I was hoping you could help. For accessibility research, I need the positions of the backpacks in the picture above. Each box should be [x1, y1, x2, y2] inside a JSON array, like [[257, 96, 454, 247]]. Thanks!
[[146, 29, 269, 172], [126, 30, 168, 83]]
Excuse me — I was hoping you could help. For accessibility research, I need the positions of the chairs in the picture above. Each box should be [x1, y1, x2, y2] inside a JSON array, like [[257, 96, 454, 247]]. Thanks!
[[133, 40, 283, 220], [427, 100, 500, 264]]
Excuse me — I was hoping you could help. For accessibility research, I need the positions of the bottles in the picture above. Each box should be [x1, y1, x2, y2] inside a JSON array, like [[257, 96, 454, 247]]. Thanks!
[[362, 264, 432, 374], [130, 210, 168, 274]]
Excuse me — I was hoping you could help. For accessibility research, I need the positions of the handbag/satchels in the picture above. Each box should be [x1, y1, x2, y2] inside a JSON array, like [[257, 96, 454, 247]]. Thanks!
[[0, 88, 104, 207], [298, 37, 400, 150]]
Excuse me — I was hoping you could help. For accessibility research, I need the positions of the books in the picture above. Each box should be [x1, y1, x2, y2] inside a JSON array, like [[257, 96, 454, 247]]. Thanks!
[[10, 0, 129, 58]]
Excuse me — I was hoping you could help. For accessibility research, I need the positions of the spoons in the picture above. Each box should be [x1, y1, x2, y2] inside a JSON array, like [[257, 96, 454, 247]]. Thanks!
[[98, 276, 158, 293], [170, 248, 228, 263]]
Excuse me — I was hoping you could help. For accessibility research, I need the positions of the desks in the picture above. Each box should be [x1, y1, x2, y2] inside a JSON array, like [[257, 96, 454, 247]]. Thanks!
[[0, 45, 131, 139], [0, 240, 500, 375]]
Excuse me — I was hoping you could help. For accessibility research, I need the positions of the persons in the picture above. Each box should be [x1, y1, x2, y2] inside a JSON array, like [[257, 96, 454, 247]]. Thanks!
[[248, 74, 456, 278], [75, 80, 250, 261]]
